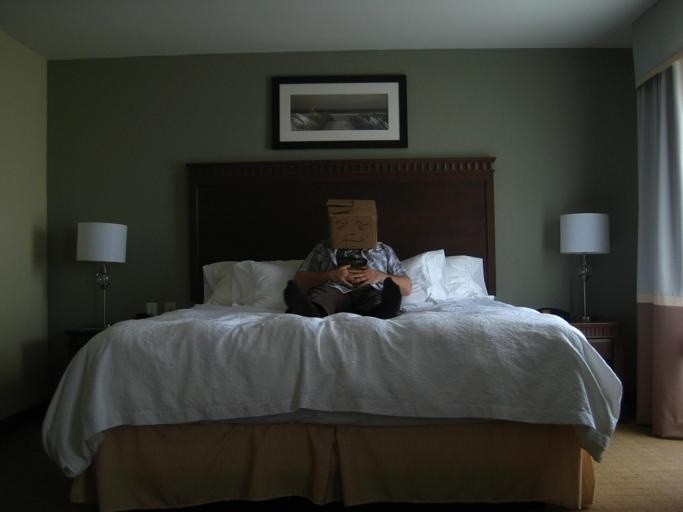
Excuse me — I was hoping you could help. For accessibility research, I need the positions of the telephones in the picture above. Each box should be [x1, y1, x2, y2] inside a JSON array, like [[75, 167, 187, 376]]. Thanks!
[[536, 308, 571, 324]]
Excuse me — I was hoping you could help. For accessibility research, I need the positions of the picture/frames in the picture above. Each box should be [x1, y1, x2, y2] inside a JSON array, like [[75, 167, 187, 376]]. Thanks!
[[269, 74, 410, 149]]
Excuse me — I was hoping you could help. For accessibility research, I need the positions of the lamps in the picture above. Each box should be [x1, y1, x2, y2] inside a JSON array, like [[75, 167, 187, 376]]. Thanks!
[[75, 220, 128, 329], [199, 250, 497, 305], [554, 211, 611, 321]]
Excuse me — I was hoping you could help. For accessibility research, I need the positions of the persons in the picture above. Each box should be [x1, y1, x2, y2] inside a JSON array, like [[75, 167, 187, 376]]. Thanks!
[[280, 227, 412, 317]]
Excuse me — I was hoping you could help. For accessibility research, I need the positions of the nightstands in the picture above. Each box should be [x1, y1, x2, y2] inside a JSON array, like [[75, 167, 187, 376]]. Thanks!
[[58, 330, 92, 382], [573, 320, 621, 380]]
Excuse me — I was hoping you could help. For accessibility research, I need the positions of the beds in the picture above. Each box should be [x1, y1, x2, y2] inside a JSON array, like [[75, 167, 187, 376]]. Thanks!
[[47, 157, 621, 512]]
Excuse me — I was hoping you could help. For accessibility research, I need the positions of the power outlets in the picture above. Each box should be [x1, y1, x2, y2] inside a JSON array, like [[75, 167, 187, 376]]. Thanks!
[[144, 303, 159, 316]]
